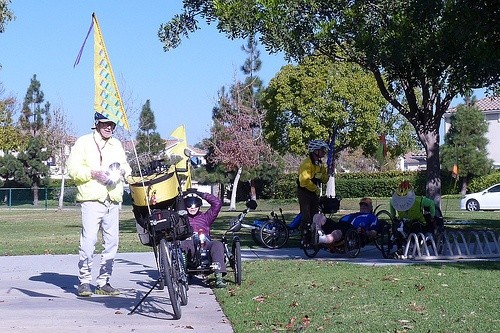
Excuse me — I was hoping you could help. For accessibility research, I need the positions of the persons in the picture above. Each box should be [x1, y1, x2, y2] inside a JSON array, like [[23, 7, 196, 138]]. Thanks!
[[385, 180, 436, 258], [296, 140, 329, 242], [66, 111, 132, 295], [177, 188, 228, 288], [301, 198, 380, 246]]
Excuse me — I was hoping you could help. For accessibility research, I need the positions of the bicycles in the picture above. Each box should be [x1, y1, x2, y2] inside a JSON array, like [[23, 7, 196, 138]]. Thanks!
[[128, 189, 191, 322]]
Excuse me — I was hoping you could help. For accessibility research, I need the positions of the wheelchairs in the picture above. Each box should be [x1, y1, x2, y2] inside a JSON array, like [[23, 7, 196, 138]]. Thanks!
[[169, 229, 242, 285]]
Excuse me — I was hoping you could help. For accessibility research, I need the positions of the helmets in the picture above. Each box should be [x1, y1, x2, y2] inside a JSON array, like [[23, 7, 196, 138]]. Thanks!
[[396, 180, 412, 196], [308, 140, 327, 152], [182, 192, 203, 210]]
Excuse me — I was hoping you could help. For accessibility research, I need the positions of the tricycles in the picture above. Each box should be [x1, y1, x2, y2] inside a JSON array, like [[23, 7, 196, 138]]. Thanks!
[[224, 198, 446, 267]]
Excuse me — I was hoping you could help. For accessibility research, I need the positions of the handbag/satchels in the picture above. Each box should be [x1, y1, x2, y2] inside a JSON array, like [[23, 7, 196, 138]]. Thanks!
[[145, 211, 174, 231]]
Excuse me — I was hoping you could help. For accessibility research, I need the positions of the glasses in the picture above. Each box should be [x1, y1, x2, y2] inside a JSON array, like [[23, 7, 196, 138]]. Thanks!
[[97, 122, 115, 131], [359, 203, 368, 207]]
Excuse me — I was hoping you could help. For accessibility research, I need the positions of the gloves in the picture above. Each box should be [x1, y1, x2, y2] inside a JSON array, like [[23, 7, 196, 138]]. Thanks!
[[182, 189, 204, 197]]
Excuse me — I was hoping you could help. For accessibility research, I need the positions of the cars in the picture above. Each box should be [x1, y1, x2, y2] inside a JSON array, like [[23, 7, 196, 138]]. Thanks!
[[460, 183, 500, 212]]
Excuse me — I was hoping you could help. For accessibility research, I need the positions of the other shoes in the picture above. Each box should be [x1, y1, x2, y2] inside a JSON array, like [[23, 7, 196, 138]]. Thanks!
[[392, 249, 403, 257], [310, 223, 319, 246]]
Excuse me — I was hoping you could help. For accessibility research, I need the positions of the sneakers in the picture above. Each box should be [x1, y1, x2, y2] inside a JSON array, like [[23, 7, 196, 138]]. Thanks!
[[95, 284, 120, 295], [78, 282, 92, 295]]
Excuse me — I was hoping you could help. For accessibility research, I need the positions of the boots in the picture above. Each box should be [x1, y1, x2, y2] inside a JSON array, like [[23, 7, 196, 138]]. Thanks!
[[214, 272, 227, 288]]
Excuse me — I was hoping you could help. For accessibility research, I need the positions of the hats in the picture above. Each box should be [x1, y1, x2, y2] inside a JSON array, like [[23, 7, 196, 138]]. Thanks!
[[391, 188, 415, 211], [359, 198, 372, 206], [95, 112, 113, 126]]
